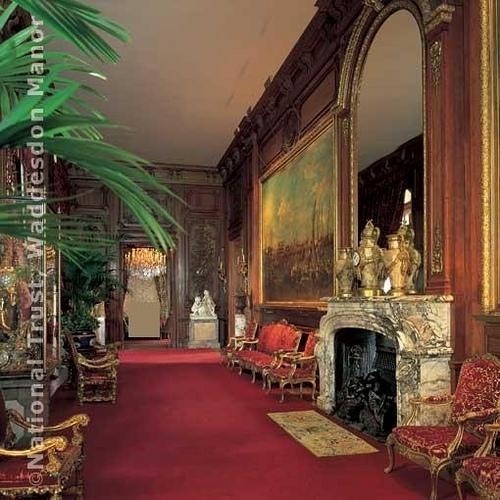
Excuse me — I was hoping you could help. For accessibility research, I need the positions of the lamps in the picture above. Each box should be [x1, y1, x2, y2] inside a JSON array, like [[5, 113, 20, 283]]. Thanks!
[[123, 247, 168, 283]]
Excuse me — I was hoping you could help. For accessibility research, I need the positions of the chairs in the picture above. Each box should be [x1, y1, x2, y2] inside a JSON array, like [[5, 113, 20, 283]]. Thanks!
[[385, 353, 499, 500], [455, 419, 500, 499], [0, 387, 90, 499], [222, 322, 257, 369], [267, 329, 317, 403], [63, 332, 118, 403]]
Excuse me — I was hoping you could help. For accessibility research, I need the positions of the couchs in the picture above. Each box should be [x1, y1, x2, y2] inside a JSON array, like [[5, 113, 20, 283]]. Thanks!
[[235, 319, 303, 390]]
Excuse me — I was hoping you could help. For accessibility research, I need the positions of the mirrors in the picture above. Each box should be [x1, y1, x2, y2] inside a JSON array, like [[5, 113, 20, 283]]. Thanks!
[[355, 9, 424, 289]]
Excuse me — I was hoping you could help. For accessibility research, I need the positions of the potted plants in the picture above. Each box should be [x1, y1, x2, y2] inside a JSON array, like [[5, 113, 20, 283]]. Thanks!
[[60, 231, 134, 348]]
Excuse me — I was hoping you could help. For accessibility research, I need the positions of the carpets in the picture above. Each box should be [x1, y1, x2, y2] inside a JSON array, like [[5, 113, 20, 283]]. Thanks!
[[267, 409, 380, 457]]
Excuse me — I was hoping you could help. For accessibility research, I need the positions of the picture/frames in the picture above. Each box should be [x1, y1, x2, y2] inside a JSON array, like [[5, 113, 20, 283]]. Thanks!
[[257, 105, 340, 308]]
